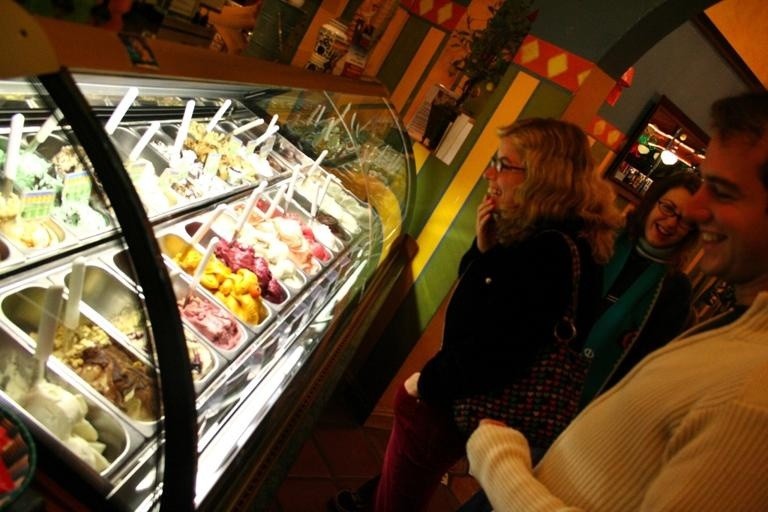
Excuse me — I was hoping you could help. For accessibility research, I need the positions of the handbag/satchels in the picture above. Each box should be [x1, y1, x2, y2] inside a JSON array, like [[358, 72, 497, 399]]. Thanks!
[[449, 338, 599, 471]]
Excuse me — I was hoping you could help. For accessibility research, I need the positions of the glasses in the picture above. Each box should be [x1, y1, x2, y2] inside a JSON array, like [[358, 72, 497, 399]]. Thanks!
[[654, 198, 694, 232], [488, 155, 527, 176]]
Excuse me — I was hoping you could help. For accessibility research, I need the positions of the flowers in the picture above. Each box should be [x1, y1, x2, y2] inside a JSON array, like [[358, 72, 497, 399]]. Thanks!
[[448, 1, 539, 109]]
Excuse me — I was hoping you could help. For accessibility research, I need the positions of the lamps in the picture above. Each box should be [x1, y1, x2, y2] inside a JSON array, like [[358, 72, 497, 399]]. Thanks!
[[603, 64, 637, 107]]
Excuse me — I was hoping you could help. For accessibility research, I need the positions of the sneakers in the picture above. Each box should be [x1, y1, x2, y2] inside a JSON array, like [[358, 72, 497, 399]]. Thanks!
[[335, 486, 368, 511]]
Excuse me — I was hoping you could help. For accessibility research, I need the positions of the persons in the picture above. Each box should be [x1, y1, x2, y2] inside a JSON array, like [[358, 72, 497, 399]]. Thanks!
[[465, 91, 768, 512], [334, 175, 702, 512], [373, 118, 626, 512]]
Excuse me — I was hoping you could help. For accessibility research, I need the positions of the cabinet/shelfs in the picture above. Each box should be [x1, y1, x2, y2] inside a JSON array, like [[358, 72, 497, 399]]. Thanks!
[[0, 0, 423, 512]]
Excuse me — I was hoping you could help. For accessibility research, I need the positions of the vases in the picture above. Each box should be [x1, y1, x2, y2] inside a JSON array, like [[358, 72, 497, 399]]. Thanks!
[[430, 81, 462, 108], [306, 16, 351, 74], [420, 101, 462, 152]]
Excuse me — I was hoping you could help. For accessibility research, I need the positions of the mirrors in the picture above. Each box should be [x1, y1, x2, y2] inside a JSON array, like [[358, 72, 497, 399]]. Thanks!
[[600, 93, 713, 209]]
